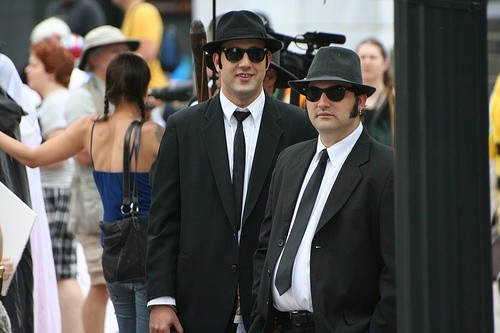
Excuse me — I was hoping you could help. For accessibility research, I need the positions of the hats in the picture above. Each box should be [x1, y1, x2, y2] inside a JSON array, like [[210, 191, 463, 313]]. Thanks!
[[30, 17, 85, 53], [286, 46, 378, 97], [203, 9, 284, 55], [80, 24, 141, 56]]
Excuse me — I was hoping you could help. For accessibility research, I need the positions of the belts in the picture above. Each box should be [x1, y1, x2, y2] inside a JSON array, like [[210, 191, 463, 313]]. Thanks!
[[271, 307, 315, 328]]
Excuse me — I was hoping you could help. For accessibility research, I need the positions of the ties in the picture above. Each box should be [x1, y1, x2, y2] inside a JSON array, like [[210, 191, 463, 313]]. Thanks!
[[275, 148, 327, 298], [228, 108, 252, 237]]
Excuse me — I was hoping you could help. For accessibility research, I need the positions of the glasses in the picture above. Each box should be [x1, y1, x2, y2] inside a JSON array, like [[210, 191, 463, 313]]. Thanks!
[[218, 46, 273, 62], [300, 84, 360, 102]]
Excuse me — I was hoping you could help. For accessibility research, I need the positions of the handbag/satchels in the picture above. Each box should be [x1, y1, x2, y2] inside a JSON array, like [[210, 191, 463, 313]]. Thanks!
[[93, 212, 153, 283]]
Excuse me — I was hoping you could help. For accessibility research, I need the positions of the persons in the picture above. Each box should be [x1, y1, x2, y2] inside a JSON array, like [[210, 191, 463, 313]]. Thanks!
[[0, 53, 164, 332], [0, 54, 62, 333], [0, 227, 12, 333], [45, 0, 107, 39], [354, 39, 397, 147], [23, 43, 85, 333], [113, 0, 169, 92], [264, 47, 301, 99], [0, 84, 34, 333], [146, 9, 320, 333], [31, 18, 72, 48], [65, 26, 142, 333], [248, 47, 398, 332]]
